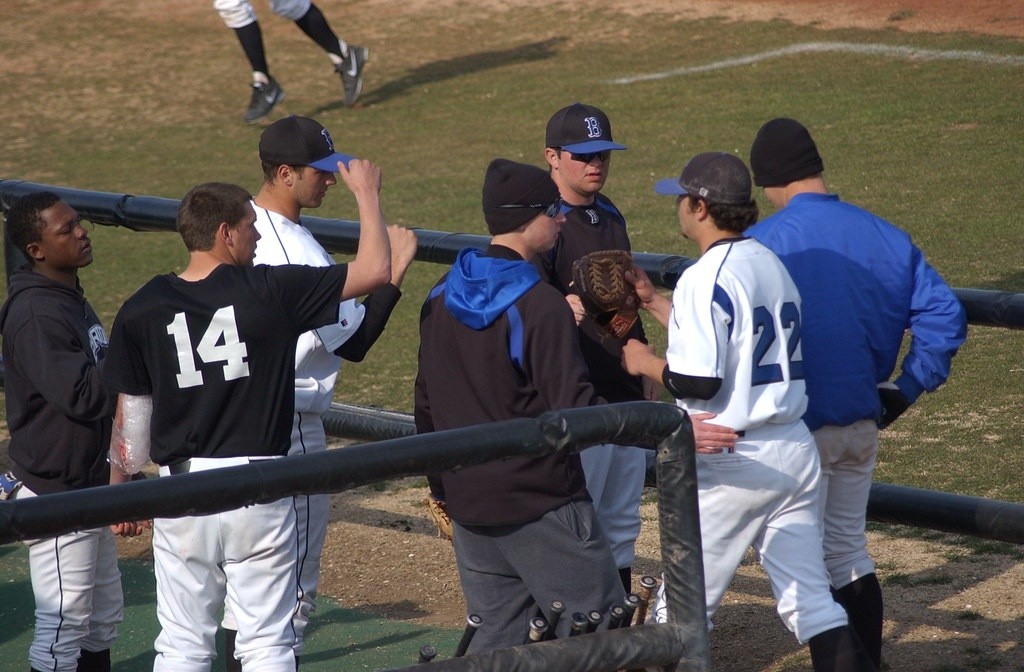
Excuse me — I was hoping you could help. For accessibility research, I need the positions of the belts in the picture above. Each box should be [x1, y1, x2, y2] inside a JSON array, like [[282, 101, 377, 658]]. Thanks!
[[169, 459, 277, 476], [734, 429, 745, 440]]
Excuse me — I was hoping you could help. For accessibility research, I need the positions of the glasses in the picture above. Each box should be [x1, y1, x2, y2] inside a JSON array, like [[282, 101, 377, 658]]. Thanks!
[[555, 149, 611, 162], [500, 197, 562, 219]]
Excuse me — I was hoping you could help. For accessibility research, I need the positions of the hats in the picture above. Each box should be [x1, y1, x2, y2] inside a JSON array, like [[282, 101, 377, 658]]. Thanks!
[[483, 158, 561, 235], [258, 115, 360, 172], [546, 102, 627, 154], [750, 118, 825, 186], [652, 152, 753, 206]]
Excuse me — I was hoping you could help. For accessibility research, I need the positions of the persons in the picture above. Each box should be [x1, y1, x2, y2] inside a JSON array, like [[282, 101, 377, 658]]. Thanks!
[[227, 115, 413, 672], [415, 156, 630, 650], [214, 0, 369, 125], [620, 152, 854, 671], [107, 158, 393, 672], [0, 191, 108, 672], [533, 104, 657, 597], [742, 118, 967, 672]]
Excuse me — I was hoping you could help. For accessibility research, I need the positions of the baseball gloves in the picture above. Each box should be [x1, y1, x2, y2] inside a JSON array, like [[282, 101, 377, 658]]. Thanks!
[[568, 249, 642, 315]]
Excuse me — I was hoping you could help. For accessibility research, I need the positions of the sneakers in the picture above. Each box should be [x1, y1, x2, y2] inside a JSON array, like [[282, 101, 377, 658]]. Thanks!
[[244, 75, 285, 124], [333, 45, 369, 108]]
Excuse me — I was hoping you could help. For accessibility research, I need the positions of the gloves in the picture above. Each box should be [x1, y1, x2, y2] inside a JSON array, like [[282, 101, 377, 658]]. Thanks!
[[877, 391, 911, 431], [0, 472, 22, 500]]
